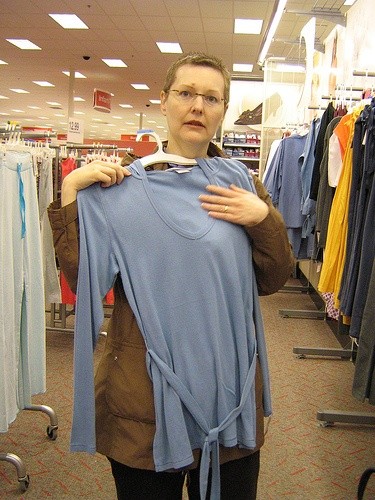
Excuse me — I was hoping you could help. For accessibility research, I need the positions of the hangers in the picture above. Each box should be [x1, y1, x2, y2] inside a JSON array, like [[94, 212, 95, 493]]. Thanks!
[[134, 129, 200, 167], [282, 72, 375, 136], [1, 121, 122, 177]]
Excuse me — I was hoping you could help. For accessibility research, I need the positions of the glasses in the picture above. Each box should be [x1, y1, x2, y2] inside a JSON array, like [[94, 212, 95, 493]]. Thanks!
[[164, 86, 225, 107]]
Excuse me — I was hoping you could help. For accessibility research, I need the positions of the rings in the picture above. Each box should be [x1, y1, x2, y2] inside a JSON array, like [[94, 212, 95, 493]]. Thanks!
[[222, 205, 230, 214]]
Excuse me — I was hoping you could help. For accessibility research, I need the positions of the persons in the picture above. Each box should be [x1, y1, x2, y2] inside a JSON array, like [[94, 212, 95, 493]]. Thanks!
[[45, 52, 295, 498]]
